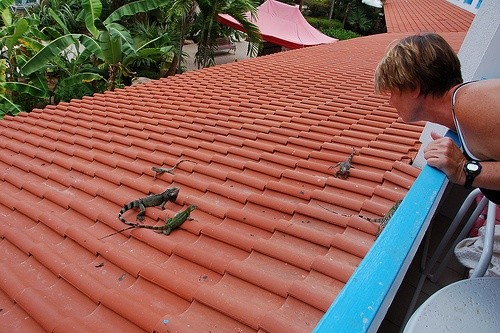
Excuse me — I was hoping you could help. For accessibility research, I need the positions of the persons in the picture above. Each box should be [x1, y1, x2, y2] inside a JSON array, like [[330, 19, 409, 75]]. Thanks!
[[373, 32, 500, 206]]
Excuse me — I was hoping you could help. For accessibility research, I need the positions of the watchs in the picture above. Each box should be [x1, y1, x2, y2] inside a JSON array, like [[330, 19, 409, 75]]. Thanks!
[[463, 160, 482, 188]]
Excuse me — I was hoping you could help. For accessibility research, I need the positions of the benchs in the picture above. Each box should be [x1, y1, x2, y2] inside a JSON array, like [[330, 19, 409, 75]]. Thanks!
[[209, 36, 237, 55]]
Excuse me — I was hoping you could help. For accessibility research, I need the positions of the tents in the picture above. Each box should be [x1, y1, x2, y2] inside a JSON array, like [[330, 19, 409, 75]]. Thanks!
[[216, 0, 339, 50]]
